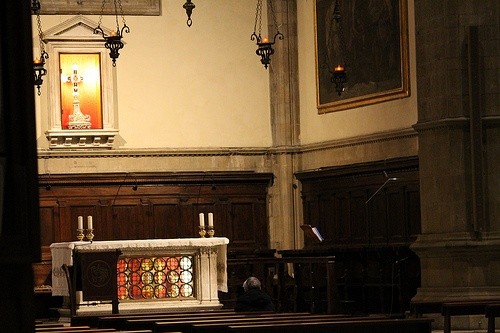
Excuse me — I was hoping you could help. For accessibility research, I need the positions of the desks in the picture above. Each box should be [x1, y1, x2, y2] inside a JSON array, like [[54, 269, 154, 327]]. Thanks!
[[49, 238, 229, 311]]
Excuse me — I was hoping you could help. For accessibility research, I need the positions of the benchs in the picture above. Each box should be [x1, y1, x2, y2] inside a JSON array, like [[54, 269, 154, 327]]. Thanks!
[[34, 302, 500, 333]]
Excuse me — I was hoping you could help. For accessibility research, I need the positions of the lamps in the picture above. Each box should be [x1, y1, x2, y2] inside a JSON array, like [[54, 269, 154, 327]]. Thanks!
[[31, 0, 50, 97], [326, 4, 348, 97], [250, 0, 284, 69], [93, 0, 130, 67]]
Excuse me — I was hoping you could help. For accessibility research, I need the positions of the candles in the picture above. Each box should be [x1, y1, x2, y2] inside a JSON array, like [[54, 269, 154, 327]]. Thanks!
[[208, 213, 214, 226], [78, 216, 83, 230], [88, 215, 92, 229], [199, 212, 204, 226]]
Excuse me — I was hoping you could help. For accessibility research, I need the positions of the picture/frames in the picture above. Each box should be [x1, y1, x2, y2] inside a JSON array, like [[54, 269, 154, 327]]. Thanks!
[[312, 0, 410, 115]]
